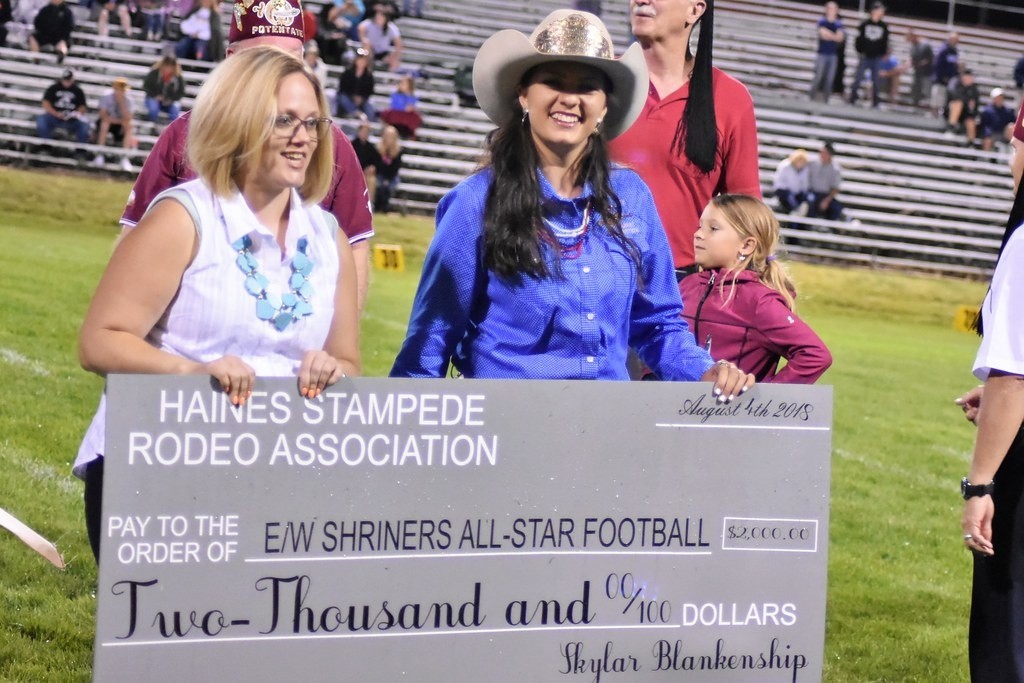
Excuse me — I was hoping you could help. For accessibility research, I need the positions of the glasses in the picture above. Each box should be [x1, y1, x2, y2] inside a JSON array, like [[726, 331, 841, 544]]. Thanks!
[[272, 113, 332, 140]]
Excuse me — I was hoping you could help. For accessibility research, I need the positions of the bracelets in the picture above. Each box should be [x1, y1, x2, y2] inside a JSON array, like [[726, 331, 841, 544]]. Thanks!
[[343, 372, 347, 377]]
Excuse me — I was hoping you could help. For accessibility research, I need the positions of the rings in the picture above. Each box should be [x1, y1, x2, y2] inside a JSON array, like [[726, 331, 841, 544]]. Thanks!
[[728, 365, 736, 368], [719, 359, 726, 365], [964, 535, 971, 538]]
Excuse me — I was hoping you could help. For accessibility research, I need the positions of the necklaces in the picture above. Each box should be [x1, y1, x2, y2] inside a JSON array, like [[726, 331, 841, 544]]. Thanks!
[[536, 201, 595, 259], [234, 233, 313, 332]]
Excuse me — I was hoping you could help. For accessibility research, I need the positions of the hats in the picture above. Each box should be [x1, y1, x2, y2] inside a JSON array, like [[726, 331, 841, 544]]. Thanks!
[[472, 7, 649, 140], [229, 0, 304, 44]]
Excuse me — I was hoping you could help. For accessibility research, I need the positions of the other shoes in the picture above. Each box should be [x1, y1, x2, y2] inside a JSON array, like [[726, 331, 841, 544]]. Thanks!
[[92, 153, 105, 169], [119, 156, 133, 172]]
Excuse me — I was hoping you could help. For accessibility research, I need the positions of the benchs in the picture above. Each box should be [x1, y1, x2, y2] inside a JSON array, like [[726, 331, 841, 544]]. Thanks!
[[0, 1, 1024, 275]]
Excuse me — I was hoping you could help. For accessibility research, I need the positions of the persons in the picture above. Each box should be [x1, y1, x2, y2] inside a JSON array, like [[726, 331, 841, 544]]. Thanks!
[[118, 0, 375, 312], [639, 194, 833, 384], [71, 47, 360, 562], [955, 49, 1024, 683], [773, 144, 843, 220], [809, 0, 1018, 153], [601, 0, 760, 381], [301, 0, 421, 209], [0, 0, 226, 172], [388, 7, 756, 402]]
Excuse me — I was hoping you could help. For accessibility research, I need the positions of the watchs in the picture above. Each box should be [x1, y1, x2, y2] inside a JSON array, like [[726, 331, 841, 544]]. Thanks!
[[961, 477, 994, 500]]
